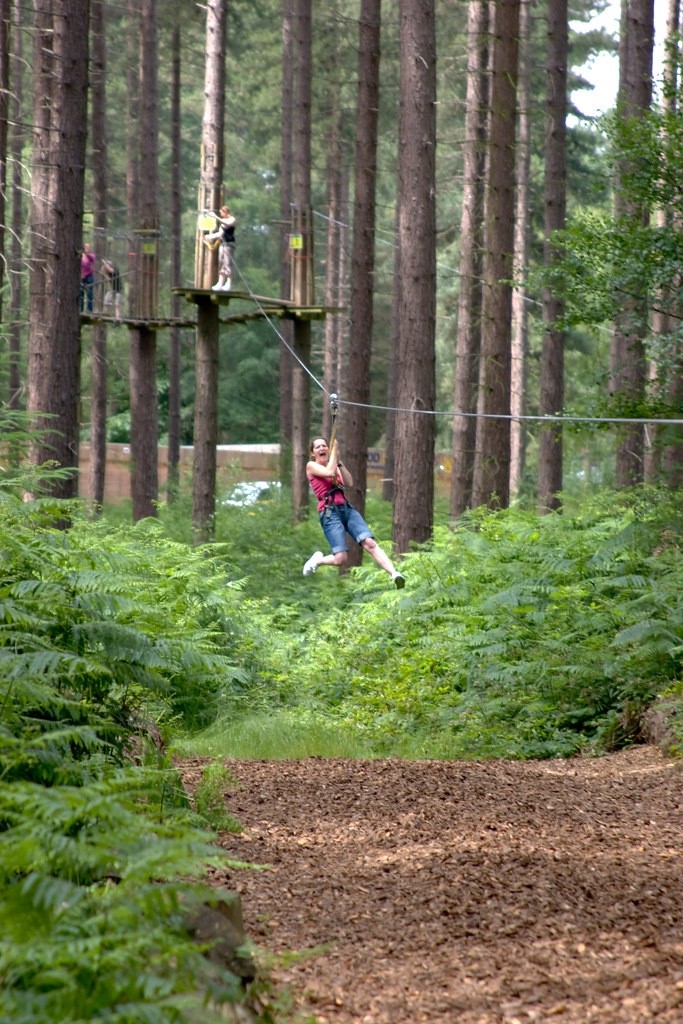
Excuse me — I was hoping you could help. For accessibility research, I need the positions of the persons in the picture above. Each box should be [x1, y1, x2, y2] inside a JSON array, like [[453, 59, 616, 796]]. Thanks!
[[206, 206, 236, 291], [304, 438, 404, 589], [79, 243, 95, 312], [101, 257, 126, 318]]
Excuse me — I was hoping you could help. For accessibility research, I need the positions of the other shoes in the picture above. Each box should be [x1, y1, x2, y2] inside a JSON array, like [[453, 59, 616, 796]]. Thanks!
[[223, 282, 230, 291], [212, 282, 223, 290], [393, 573, 405, 590], [303, 551, 323, 577]]
